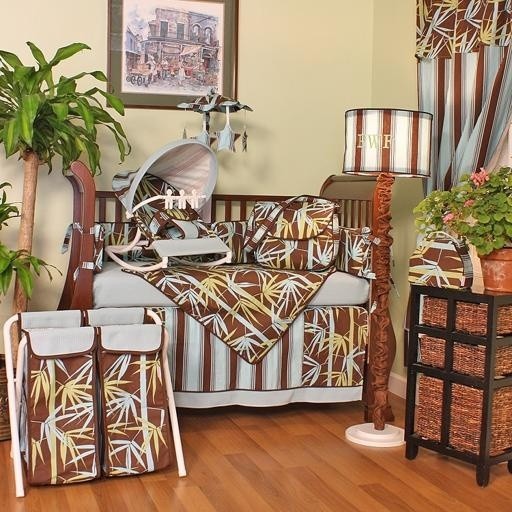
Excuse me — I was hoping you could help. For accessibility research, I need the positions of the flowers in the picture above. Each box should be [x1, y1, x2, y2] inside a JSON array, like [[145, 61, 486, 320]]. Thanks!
[[416, 164, 511, 255]]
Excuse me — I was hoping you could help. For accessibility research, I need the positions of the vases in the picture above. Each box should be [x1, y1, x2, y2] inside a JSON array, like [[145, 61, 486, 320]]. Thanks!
[[477, 245, 511, 292]]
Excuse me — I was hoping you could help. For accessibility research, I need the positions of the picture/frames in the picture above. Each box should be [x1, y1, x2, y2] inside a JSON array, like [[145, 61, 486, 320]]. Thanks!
[[106, 1, 241, 112]]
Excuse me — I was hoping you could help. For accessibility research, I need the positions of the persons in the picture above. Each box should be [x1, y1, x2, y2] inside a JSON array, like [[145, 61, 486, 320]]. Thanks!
[[146, 55, 186, 85]]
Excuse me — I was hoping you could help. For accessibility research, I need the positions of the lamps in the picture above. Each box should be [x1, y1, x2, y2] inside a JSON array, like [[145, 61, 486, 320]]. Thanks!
[[344, 109, 433, 451]]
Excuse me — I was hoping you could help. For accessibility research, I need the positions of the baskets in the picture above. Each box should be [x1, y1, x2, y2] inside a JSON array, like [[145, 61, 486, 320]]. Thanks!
[[423, 297, 512, 335], [416, 374, 511, 456], [418, 335, 512, 377]]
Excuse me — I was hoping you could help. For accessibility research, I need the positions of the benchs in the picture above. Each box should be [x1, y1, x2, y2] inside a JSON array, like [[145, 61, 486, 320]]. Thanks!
[[56, 160, 394, 432]]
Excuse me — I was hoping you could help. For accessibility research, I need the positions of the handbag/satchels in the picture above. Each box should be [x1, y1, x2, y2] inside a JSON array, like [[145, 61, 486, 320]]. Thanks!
[[244, 195, 340, 272], [408, 231, 473, 290]]
